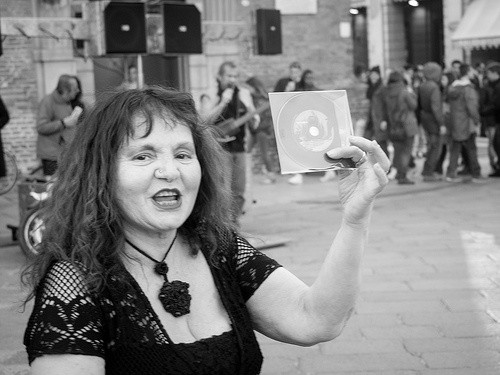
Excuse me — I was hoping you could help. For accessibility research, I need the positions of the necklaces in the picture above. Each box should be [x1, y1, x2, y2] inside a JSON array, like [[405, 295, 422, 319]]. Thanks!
[[118, 229, 193, 318]]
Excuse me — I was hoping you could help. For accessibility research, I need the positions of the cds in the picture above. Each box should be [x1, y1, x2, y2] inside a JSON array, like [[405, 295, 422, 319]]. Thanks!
[[277, 93, 351, 170]]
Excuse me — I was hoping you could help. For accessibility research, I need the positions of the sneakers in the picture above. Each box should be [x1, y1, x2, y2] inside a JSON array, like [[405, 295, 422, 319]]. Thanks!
[[446, 176, 464, 184], [423, 174, 443, 182], [320, 169, 337, 182], [287, 173, 303, 185], [398, 176, 415, 185], [471, 177, 488, 185]]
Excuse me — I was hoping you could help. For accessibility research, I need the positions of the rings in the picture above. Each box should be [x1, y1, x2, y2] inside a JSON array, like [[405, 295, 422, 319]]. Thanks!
[[355, 152, 367, 168]]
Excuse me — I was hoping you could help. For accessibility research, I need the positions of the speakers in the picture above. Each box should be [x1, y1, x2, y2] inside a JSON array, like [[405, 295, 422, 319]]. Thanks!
[[163, 3, 202, 54], [103, 1, 146, 54], [257, 9, 281, 55]]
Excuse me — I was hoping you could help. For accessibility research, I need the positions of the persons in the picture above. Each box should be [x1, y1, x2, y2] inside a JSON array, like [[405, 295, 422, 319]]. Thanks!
[[20, 87, 391, 375], [403, 59, 500, 165], [296, 70, 318, 91], [117, 66, 147, 91], [275, 62, 302, 92], [201, 62, 260, 217], [418, 61, 449, 181], [361, 63, 393, 170], [385, 70, 417, 184], [446, 63, 489, 184], [36, 74, 82, 177], [485, 63, 499, 176]]
